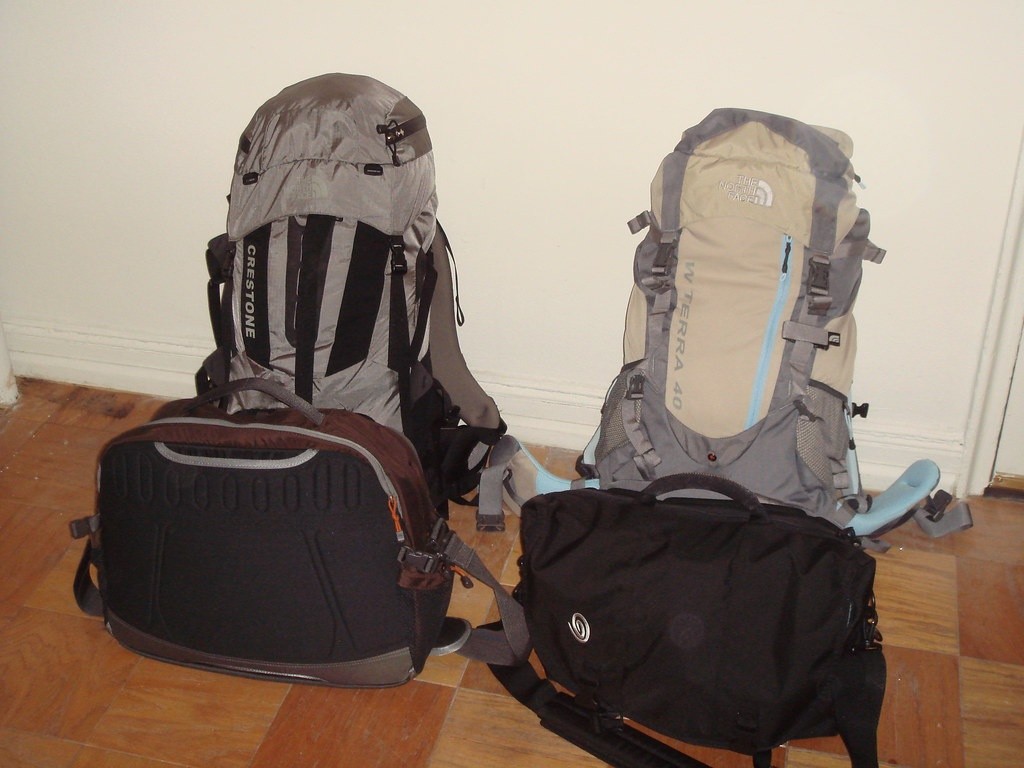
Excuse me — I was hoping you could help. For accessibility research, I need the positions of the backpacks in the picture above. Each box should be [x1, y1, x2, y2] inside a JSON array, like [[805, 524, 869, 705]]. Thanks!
[[476, 106, 973, 551], [195, 72, 506, 505]]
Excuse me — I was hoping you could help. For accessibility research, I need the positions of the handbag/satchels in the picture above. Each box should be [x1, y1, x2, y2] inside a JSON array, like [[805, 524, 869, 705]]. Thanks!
[[68, 378, 531, 688], [476, 474, 886, 768]]
